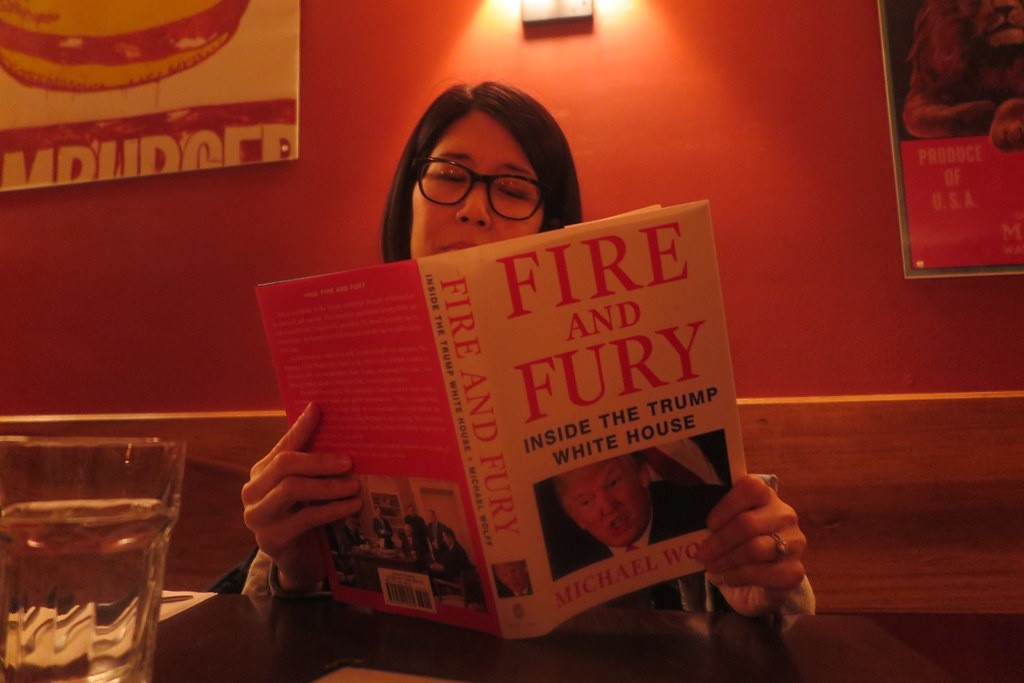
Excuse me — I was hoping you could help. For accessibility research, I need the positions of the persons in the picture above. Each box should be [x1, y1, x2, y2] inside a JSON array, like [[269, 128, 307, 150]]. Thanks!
[[492, 562, 533, 599], [547, 455, 730, 583], [209, 80, 815, 618], [334, 504, 483, 604]]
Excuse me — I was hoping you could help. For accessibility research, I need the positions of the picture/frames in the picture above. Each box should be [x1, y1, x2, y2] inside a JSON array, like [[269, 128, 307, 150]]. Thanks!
[[877, 0, 1024, 278]]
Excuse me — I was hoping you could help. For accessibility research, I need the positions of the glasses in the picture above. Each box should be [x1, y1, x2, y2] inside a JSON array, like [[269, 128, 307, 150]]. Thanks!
[[415, 157, 545, 221]]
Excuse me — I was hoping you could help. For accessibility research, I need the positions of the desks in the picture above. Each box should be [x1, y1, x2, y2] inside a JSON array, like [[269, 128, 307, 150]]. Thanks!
[[146, 591, 1024, 683]]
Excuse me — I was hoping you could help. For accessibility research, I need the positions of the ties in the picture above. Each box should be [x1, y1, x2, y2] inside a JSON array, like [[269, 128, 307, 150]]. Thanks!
[[408, 539, 411, 550], [433, 525, 437, 539]]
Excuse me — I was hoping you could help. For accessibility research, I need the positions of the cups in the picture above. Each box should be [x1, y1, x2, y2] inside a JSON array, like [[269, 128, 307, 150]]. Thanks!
[[0, 433, 187, 683]]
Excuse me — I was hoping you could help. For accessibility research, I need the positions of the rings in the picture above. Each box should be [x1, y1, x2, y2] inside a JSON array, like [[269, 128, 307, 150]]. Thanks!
[[770, 531, 791, 562]]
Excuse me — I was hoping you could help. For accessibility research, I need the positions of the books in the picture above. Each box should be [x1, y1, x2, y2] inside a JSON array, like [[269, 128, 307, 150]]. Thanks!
[[255, 202, 749, 639]]
